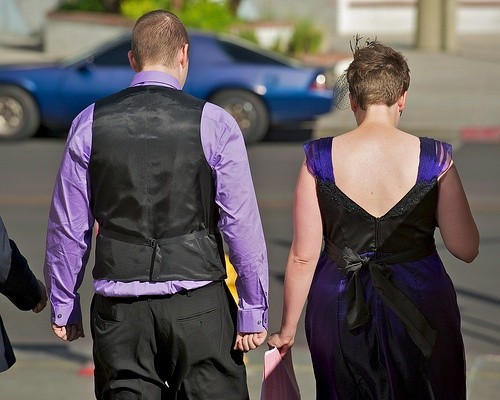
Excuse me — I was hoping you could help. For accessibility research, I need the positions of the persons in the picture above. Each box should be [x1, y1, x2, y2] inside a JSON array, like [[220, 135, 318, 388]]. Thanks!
[[0, 216, 49, 373], [267, 35, 480, 400], [44, 9, 269, 400]]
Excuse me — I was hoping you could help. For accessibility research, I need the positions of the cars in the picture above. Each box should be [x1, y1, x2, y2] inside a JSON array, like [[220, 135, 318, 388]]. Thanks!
[[0, 30, 336, 145]]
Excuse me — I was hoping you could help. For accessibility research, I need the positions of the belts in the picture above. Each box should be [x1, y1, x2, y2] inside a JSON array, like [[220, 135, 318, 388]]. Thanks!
[[320, 237, 437, 274]]
